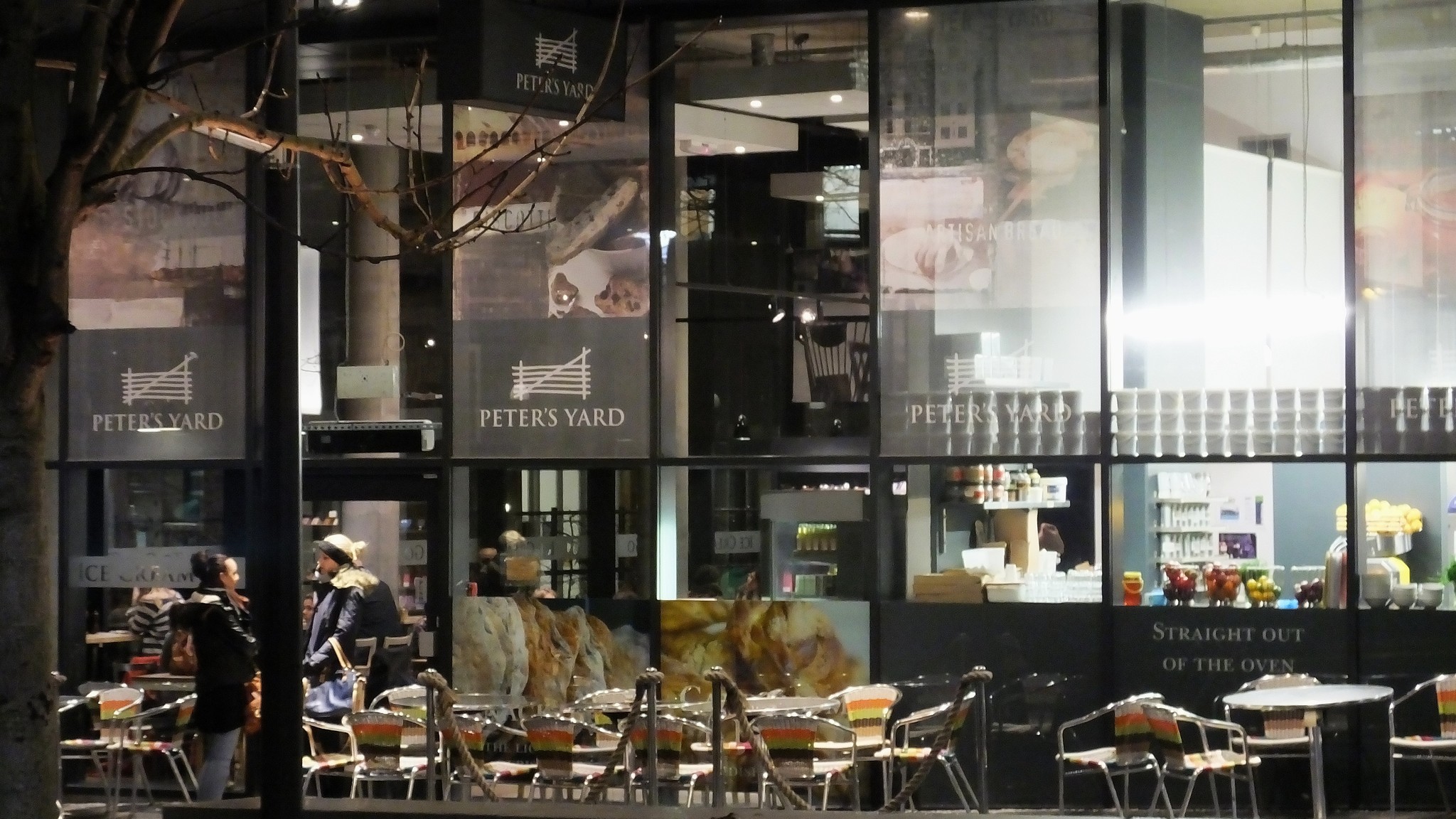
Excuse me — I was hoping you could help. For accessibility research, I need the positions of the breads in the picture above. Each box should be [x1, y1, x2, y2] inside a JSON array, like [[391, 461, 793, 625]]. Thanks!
[[659, 601, 867, 713], [449, 597, 655, 710]]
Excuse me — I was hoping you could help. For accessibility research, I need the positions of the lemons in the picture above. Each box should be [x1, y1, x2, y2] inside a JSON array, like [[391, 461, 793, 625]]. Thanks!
[[1337, 499, 1423, 533], [1247, 576, 1282, 602]]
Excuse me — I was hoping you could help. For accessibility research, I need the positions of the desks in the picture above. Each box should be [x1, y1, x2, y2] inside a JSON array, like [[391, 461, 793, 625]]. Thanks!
[[1223, 684, 1394, 819], [682, 694, 842, 808], [389, 689, 527, 799], [561, 698, 693, 805]]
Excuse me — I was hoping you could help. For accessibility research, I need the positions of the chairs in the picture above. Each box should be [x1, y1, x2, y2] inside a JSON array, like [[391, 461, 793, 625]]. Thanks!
[[1053, 692, 1176, 819], [303, 633, 979, 816], [56, 690, 198, 819], [1142, 703, 1261, 819], [1388, 674, 1456, 819], [1215, 670, 1324, 819]]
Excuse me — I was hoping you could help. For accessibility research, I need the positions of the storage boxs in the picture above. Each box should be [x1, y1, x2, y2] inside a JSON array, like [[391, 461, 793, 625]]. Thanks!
[[912, 574, 981, 603]]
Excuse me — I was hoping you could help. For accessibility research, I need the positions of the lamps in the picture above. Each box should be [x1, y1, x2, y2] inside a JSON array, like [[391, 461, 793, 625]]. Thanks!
[[732, 416, 752, 441], [767, 295, 786, 323]]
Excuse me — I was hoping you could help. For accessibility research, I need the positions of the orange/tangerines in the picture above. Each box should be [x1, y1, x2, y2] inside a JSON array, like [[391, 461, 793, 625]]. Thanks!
[[1205, 577, 1240, 602]]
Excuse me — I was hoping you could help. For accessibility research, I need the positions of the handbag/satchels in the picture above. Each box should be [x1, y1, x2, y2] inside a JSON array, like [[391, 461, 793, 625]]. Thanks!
[[242, 671, 261, 735], [304, 636, 361, 718]]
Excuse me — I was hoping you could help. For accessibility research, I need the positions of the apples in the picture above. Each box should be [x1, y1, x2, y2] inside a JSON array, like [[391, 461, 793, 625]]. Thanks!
[[1205, 564, 1238, 587], [1162, 566, 1196, 601]]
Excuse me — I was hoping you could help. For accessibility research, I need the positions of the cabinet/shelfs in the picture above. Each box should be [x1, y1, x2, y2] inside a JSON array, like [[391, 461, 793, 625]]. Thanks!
[[1156, 498, 1217, 567]]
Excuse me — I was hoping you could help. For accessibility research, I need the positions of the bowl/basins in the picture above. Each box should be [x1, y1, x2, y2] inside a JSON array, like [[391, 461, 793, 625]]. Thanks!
[[1361, 573, 1445, 609], [1161, 564, 1325, 608]]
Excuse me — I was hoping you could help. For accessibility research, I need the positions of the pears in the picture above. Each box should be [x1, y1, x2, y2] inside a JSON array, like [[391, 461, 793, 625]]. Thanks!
[[1295, 581, 1324, 603]]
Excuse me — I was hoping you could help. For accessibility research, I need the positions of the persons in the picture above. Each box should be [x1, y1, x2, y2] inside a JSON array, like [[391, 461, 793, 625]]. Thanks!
[[187, 554, 258, 802], [477, 530, 558, 598], [303, 534, 403, 751], [123, 565, 196, 734]]
